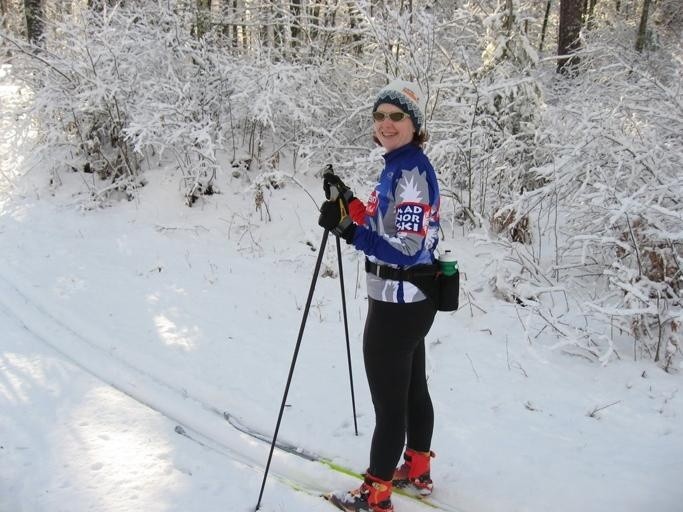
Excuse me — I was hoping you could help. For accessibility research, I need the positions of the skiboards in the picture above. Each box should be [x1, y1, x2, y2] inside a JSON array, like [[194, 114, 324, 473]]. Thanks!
[[175, 411, 456, 512]]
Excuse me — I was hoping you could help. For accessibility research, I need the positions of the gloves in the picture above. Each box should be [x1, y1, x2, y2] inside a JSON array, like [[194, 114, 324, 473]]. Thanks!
[[318, 194, 356, 244], [322, 164, 356, 205]]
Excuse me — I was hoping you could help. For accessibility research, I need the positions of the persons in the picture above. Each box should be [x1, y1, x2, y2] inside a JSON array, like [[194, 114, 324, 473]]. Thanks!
[[315, 80, 443, 512]]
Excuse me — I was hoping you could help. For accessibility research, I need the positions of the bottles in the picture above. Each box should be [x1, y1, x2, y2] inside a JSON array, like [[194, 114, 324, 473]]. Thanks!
[[437, 249, 461, 277]]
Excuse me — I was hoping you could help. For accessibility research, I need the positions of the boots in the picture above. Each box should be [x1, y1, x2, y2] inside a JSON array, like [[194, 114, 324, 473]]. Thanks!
[[327, 469, 393, 512], [392, 447, 433, 497]]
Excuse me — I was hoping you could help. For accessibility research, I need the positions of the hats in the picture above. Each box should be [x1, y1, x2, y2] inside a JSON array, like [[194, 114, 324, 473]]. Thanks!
[[373, 80, 424, 130]]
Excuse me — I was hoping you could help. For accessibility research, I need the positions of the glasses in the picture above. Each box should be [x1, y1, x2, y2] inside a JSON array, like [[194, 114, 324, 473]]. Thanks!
[[372, 112, 410, 120]]
